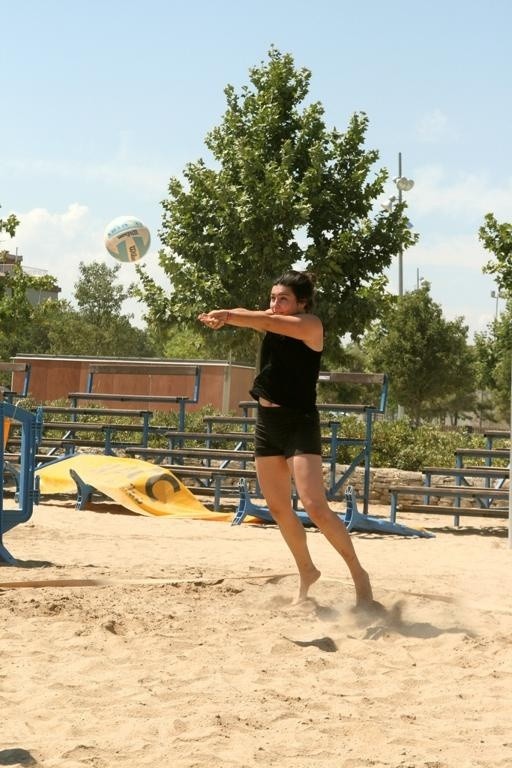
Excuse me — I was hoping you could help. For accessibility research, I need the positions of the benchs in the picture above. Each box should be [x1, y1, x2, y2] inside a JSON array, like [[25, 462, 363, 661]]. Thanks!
[[388, 427, 509, 527], [0, 368, 389, 511]]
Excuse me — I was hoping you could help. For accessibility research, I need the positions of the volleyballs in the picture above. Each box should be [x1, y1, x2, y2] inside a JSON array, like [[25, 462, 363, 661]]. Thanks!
[[104, 215, 149, 262]]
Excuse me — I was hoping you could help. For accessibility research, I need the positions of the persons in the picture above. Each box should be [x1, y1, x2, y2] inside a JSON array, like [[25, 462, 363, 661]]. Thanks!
[[197, 269, 373, 609]]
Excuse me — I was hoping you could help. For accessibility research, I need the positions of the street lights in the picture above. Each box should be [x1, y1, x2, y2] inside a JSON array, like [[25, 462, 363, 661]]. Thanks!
[[374, 152, 415, 304], [417, 267, 432, 289]]
